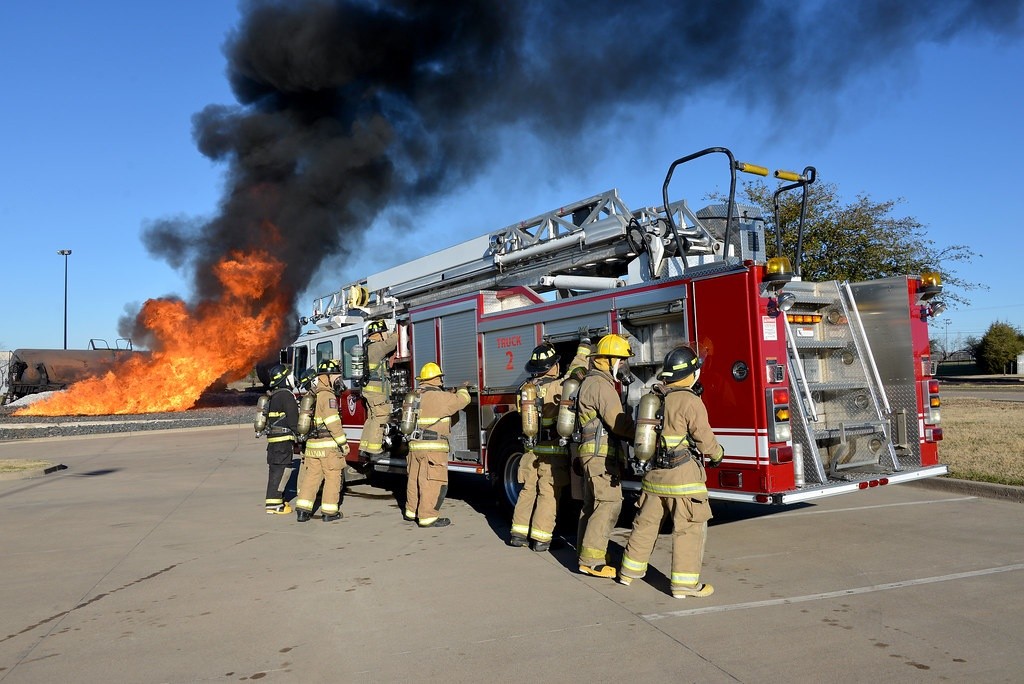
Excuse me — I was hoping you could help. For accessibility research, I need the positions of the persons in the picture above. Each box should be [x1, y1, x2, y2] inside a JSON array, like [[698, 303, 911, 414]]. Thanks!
[[253, 378, 257, 387], [618, 346, 724, 599], [405, 362, 472, 528], [265, 359, 350, 522], [510, 325, 590, 552], [358, 319, 400, 461], [578, 334, 635, 578]]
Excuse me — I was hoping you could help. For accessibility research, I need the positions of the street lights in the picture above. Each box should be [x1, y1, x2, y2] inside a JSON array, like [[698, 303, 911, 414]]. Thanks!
[[56, 249, 72, 350]]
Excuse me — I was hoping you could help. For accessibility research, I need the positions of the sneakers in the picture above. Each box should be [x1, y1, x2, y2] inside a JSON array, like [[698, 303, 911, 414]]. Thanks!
[[672, 584, 715, 598], [265, 506, 292, 514], [619, 572, 633, 586], [579, 564, 616, 577]]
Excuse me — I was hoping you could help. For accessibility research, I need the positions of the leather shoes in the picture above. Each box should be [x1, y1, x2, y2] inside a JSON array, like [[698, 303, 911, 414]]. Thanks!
[[297, 511, 310, 522], [370, 452, 390, 461], [359, 450, 369, 457], [322, 512, 343, 521], [510, 535, 529, 547], [418, 517, 451, 527], [405, 515, 418, 520], [533, 541, 551, 552]]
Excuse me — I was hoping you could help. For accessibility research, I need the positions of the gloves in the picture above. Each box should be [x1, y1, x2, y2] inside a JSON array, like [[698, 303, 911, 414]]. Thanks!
[[578, 326, 592, 345], [710, 444, 724, 467]]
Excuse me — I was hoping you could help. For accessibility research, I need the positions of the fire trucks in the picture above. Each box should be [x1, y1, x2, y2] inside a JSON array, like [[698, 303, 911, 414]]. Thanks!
[[279, 147, 949, 538]]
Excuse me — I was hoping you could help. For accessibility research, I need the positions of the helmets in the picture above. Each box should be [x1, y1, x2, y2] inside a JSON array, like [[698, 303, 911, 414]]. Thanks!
[[317, 360, 342, 374], [524, 343, 561, 374], [362, 321, 389, 337], [586, 333, 633, 357], [269, 364, 291, 388], [657, 346, 702, 381], [301, 369, 318, 388], [415, 363, 446, 380]]
